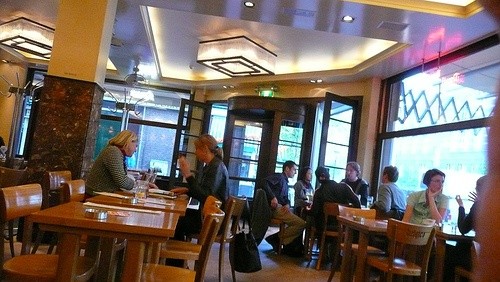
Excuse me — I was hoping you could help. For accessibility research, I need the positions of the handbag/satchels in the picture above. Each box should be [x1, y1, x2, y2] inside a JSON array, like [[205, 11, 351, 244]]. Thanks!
[[229, 195, 262, 273]]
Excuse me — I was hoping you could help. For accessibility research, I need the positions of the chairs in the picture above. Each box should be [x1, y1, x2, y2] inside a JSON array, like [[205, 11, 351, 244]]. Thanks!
[[251, 189, 285, 254], [0, 183, 97, 282], [38, 171, 128, 277], [139, 207, 226, 282], [325, 206, 385, 282], [363, 218, 438, 282], [0, 154, 29, 189], [454, 237, 485, 282], [185, 195, 247, 281], [159, 195, 222, 267], [308, 203, 348, 271]]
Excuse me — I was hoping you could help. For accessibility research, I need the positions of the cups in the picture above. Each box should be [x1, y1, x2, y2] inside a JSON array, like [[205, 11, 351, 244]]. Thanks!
[[306, 189, 314, 200], [135, 180, 149, 203], [367, 196, 373, 209]]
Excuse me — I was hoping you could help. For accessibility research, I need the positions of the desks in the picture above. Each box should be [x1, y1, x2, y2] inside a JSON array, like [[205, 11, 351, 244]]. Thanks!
[[431, 226, 477, 282], [87, 189, 190, 263], [29, 199, 181, 282], [335, 215, 388, 281]]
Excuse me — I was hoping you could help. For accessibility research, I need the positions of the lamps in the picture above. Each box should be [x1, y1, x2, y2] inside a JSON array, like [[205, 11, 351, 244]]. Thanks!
[[253, 85, 278, 98], [196, 29, 281, 78], [0, 16, 56, 61]]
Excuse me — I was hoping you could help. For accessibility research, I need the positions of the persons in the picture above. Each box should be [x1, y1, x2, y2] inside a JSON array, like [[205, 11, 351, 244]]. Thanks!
[[443, 174, 487, 282], [339, 162, 368, 208], [260, 161, 306, 253], [0, 136, 8, 166], [309, 166, 361, 266], [293, 166, 311, 249], [83, 128, 140, 199], [163, 135, 231, 269], [400, 168, 450, 282], [371, 166, 406, 221]]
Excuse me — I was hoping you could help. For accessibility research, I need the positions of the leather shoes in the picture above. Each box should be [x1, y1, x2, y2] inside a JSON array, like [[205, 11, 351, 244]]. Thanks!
[[265, 235, 279, 253]]
